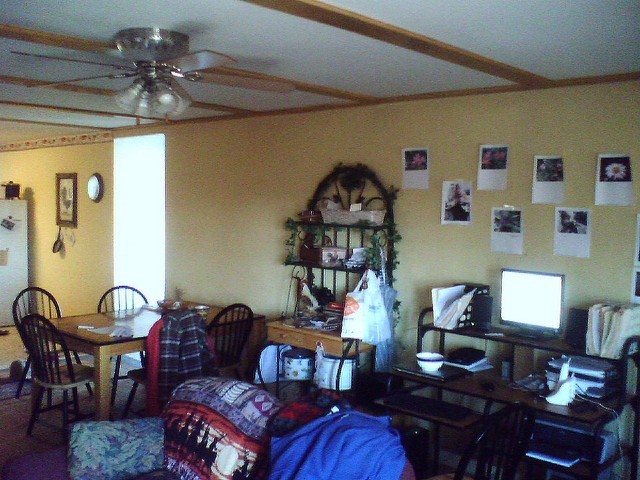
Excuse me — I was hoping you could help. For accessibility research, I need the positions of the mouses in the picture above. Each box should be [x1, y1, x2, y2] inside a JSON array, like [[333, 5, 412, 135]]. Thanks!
[[485, 381, 495, 393]]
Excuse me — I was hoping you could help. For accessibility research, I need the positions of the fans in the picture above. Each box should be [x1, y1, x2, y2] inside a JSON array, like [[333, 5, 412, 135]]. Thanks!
[[11, 29, 295, 93]]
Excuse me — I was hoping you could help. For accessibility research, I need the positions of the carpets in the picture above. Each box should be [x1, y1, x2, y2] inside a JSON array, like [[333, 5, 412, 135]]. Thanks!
[[0, 355, 148, 462]]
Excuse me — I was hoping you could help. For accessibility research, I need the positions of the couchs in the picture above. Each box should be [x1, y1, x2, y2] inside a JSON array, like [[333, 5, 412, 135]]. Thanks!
[[69, 375, 416, 480]]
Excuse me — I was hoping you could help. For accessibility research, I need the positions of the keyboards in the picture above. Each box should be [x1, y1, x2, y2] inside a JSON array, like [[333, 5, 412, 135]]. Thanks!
[[384, 392, 473, 420]]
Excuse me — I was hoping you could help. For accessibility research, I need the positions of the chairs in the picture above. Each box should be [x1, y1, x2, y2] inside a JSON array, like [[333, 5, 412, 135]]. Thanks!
[[20, 314, 115, 443], [15, 286, 92, 407], [207, 303, 253, 382], [426, 403, 535, 480], [99, 285, 149, 406], [121, 318, 213, 419]]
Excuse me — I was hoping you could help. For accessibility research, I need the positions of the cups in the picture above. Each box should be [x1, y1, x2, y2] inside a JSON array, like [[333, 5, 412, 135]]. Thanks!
[[195, 305, 210, 322]]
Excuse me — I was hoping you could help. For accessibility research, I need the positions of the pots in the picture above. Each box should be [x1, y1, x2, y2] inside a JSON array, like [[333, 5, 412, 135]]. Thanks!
[[52, 225, 63, 253], [1, 181, 20, 197]]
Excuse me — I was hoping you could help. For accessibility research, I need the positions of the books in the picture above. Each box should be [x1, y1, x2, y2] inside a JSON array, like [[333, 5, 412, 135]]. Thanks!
[[443, 346, 487, 370], [524, 444, 580, 468]]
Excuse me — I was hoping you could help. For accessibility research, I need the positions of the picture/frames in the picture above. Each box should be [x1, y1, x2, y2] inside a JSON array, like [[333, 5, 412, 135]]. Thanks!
[[56, 173, 77, 228]]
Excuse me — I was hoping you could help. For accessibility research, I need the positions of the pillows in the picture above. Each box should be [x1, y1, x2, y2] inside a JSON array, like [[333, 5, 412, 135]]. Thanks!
[[67, 417, 164, 480]]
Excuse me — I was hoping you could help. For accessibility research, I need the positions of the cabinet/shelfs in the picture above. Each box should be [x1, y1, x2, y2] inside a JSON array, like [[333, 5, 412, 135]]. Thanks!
[[286, 219, 391, 275]]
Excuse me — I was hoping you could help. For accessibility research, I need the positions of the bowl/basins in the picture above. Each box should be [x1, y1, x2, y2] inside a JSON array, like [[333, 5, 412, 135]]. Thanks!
[[416, 351, 444, 372]]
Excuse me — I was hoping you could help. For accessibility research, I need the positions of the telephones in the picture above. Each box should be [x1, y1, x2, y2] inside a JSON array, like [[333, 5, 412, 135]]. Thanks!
[[545, 357, 576, 406]]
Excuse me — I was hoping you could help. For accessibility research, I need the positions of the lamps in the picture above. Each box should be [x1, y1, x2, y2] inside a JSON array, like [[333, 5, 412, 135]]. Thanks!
[[116, 67, 196, 118]]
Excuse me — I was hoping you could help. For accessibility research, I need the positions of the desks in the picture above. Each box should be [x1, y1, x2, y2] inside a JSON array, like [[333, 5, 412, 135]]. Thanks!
[[31, 301, 265, 421], [376, 306, 640, 480], [266, 317, 375, 406]]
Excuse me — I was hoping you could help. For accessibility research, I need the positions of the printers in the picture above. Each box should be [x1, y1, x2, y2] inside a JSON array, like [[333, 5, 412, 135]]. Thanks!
[[525, 418, 617, 468]]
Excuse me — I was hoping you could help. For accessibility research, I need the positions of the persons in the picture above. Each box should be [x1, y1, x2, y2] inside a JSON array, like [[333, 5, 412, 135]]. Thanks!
[[444, 184, 469, 219]]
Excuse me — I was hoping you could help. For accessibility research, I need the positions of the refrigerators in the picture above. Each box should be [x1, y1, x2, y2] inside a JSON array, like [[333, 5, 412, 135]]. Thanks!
[[0, 199, 28, 326]]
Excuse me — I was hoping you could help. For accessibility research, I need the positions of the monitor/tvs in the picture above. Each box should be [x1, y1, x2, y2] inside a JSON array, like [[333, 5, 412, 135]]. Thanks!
[[500, 268, 567, 341]]
